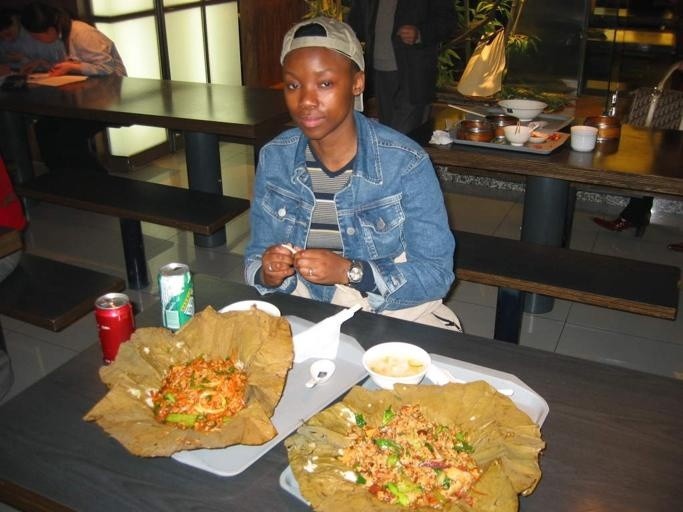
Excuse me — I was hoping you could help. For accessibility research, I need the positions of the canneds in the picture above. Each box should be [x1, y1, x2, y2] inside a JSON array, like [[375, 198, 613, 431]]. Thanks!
[[95, 293, 134, 366], [156, 261, 193, 331]]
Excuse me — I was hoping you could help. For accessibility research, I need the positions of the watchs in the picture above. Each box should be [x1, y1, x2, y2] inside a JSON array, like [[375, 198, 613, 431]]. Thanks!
[[345, 259, 364, 285]]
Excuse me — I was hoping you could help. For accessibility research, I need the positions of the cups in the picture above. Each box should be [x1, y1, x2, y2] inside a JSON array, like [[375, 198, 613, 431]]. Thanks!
[[570, 126, 598, 151]]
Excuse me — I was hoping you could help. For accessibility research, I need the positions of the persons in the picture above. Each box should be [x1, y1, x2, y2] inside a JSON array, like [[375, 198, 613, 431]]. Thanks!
[[0, 154, 29, 399], [18, 5, 127, 177], [0, 11, 67, 73], [243, 15, 463, 334], [349, 0, 458, 136], [592, 62, 683, 254]]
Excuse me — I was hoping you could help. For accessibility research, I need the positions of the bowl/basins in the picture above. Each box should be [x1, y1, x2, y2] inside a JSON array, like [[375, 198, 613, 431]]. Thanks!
[[362, 343, 430, 387], [3, 73, 27, 89], [457, 120, 493, 140], [498, 100, 548, 121], [584, 116, 622, 141], [502, 125, 532, 146], [219, 299, 281, 320], [484, 114, 518, 138]]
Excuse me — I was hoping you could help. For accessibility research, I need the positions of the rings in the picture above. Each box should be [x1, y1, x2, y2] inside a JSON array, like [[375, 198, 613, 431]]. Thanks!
[[306, 266, 313, 277], [267, 263, 273, 271]]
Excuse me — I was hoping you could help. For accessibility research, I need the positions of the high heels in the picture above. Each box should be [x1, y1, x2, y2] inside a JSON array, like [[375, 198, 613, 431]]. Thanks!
[[593, 211, 651, 238], [667, 243, 683, 252]]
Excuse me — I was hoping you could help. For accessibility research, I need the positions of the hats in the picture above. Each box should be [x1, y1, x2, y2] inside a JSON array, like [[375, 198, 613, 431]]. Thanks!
[[280, 16, 365, 73]]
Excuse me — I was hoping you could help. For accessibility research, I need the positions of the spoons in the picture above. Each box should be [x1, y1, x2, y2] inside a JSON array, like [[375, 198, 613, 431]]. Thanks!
[[306, 360, 336, 388]]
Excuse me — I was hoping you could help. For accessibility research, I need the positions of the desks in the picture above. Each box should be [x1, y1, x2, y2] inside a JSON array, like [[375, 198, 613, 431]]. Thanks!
[[0, 75, 288, 248]]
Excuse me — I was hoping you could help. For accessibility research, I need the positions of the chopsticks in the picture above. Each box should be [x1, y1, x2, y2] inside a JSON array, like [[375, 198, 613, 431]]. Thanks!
[[447, 104, 488, 118], [514, 120, 522, 134]]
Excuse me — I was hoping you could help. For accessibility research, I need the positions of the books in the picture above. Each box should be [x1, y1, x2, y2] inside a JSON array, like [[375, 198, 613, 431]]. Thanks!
[[23, 71, 88, 87]]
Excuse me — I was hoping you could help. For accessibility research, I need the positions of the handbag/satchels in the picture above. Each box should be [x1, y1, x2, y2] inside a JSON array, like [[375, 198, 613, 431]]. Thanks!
[[621, 87, 683, 131]]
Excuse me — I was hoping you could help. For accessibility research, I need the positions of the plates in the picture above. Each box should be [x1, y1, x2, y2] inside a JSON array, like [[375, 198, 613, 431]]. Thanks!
[[529, 134, 550, 143]]
